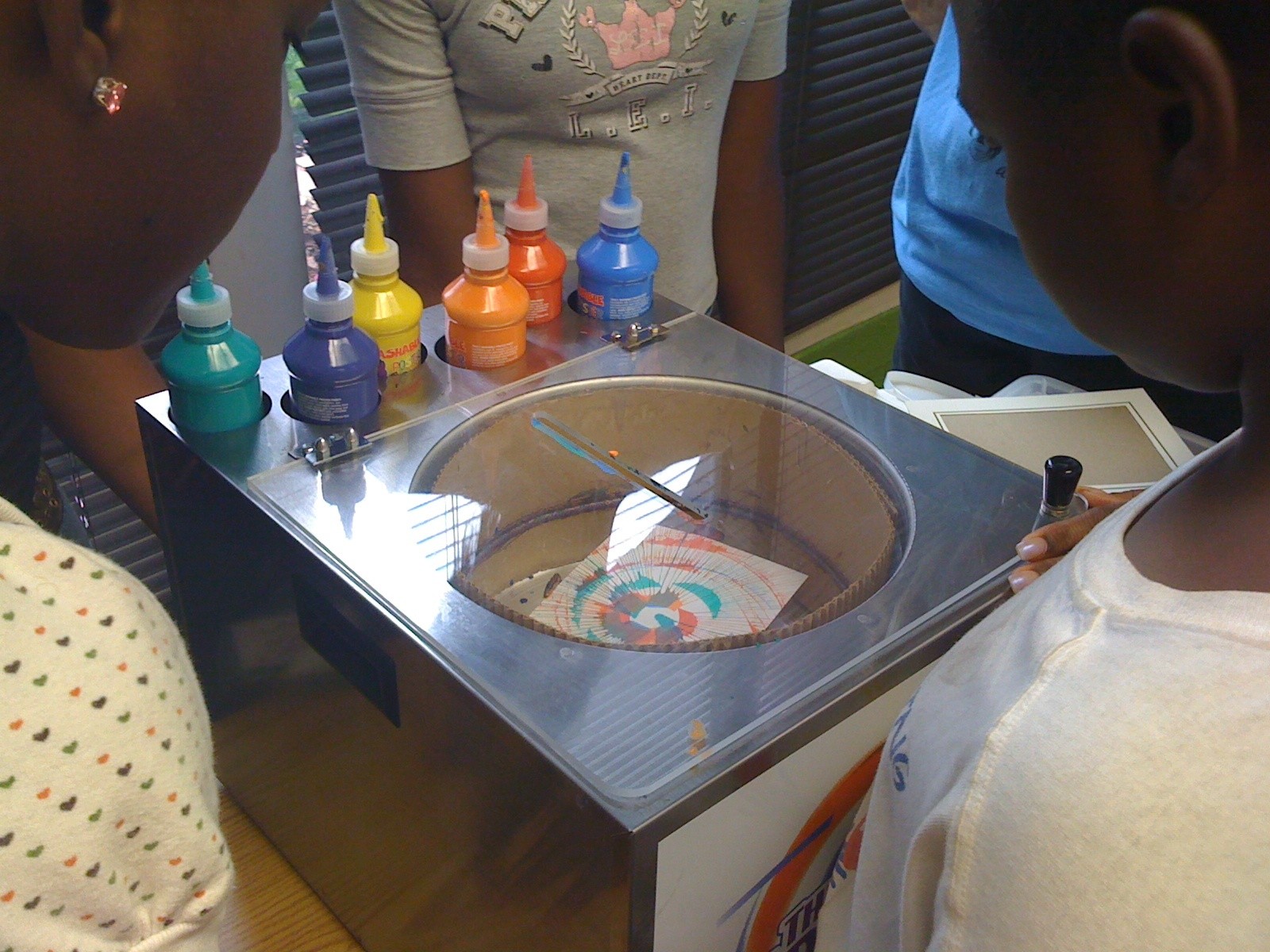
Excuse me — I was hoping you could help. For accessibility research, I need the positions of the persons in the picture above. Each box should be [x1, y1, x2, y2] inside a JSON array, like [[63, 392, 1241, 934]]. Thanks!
[[849, 0, 1270, 952], [0, 0, 329, 952], [331, 0, 793, 355]]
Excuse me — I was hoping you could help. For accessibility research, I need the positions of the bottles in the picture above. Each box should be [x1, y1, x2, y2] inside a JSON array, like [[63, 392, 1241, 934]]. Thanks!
[[158, 260, 265, 433], [346, 193, 423, 377], [501, 153, 567, 326], [441, 189, 530, 371], [281, 235, 378, 427], [576, 151, 660, 321]]
